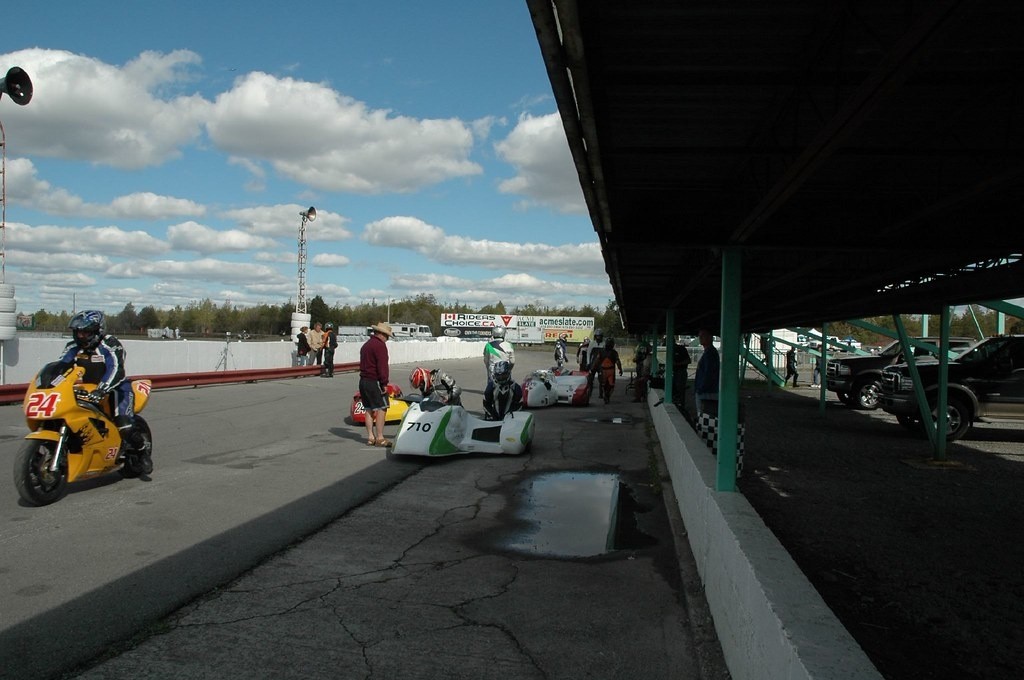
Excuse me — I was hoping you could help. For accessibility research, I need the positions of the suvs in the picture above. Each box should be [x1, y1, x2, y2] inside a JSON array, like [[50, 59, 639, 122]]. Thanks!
[[825, 336, 979, 411], [875, 331, 1024, 443]]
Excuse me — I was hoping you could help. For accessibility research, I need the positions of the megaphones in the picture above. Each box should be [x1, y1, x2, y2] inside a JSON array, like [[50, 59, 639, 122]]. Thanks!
[[0, 66, 33, 105], [299, 207, 316, 221]]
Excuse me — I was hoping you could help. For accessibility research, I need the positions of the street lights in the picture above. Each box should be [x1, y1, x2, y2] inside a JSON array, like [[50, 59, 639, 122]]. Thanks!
[[386, 297, 397, 324]]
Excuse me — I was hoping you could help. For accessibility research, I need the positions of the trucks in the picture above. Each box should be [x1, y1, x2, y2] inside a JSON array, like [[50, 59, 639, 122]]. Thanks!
[[384, 320, 433, 338], [337, 325, 374, 337]]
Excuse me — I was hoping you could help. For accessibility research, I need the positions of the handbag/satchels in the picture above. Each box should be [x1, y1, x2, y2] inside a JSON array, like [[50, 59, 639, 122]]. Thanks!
[[649, 376, 673, 389]]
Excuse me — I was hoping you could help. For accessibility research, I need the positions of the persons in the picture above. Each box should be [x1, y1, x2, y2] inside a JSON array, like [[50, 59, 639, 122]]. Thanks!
[[811, 345, 828, 388], [576, 337, 590, 372], [588, 338, 622, 404], [297, 326, 311, 378], [306, 322, 325, 377], [694, 325, 719, 418], [359, 322, 391, 447], [760, 335, 775, 363], [175, 326, 180, 339], [409, 366, 463, 406], [662, 336, 691, 405], [61, 310, 154, 474], [586, 328, 606, 399], [481, 361, 524, 421], [319, 322, 335, 378], [633, 345, 659, 403], [785, 347, 800, 387], [162, 326, 170, 338], [554, 332, 568, 370], [484, 326, 515, 387]]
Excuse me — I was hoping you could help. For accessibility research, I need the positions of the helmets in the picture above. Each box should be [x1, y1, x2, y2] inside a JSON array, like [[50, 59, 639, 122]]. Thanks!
[[559, 332, 569, 342], [635, 341, 652, 356], [324, 322, 334, 331], [67, 310, 107, 350], [494, 361, 511, 385], [593, 328, 604, 341], [605, 337, 615, 347], [490, 326, 507, 342], [410, 367, 431, 394]]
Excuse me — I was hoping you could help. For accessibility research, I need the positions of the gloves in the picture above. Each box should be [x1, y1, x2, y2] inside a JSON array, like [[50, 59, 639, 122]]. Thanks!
[[87, 382, 112, 404]]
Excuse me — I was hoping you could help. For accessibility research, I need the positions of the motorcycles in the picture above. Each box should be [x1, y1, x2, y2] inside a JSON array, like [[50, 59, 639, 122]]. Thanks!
[[12, 361, 154, 507]]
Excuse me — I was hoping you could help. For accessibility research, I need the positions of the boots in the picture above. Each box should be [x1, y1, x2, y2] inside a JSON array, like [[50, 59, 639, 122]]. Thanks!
[[136, 446, 153, 474]]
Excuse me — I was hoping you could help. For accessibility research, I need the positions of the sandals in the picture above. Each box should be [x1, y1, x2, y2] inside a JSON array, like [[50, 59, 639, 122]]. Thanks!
[[367, 438, 376, 446], [374, 438, 392, 447]]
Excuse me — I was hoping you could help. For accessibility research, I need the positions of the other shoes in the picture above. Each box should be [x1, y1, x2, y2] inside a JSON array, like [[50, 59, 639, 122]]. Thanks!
[[603, 395, 610, 404], [321, 373, 333, 378], [819, 385, 822, 389], [811, 384, 818, 388], [792, 384, 800, 387], [631, 396, 646, 403]]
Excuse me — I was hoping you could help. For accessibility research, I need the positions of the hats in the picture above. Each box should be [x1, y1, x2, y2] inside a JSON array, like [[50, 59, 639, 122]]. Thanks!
[[370, 323, 395, 339]]
